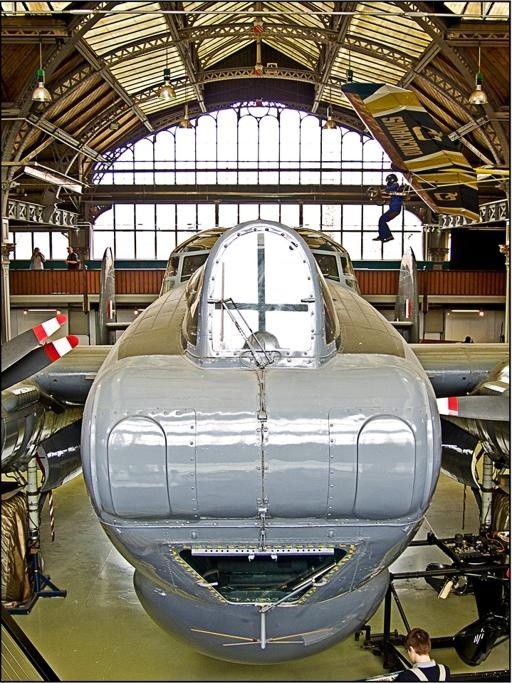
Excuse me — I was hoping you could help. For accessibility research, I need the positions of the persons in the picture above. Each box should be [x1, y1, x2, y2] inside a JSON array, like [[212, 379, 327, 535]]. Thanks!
[[394, 628, 451, 683], [66, 246, 82, 270], [28, 247, 46, 270], [371, 175, 402, 241]]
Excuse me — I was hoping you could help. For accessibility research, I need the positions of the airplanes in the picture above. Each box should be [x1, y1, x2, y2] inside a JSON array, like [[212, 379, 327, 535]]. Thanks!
[[0, 216, 510, 683], [340, 80, 509, 224]]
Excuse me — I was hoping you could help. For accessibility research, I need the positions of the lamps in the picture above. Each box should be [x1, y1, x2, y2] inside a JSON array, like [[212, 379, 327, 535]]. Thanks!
[[340, 14, 356, 83], [468, 21, 489, 104], [156, 41, 178, 98], [320, 86, 338, 129], [29, 37, 55, 102], [178, 64, 194, 127]]
